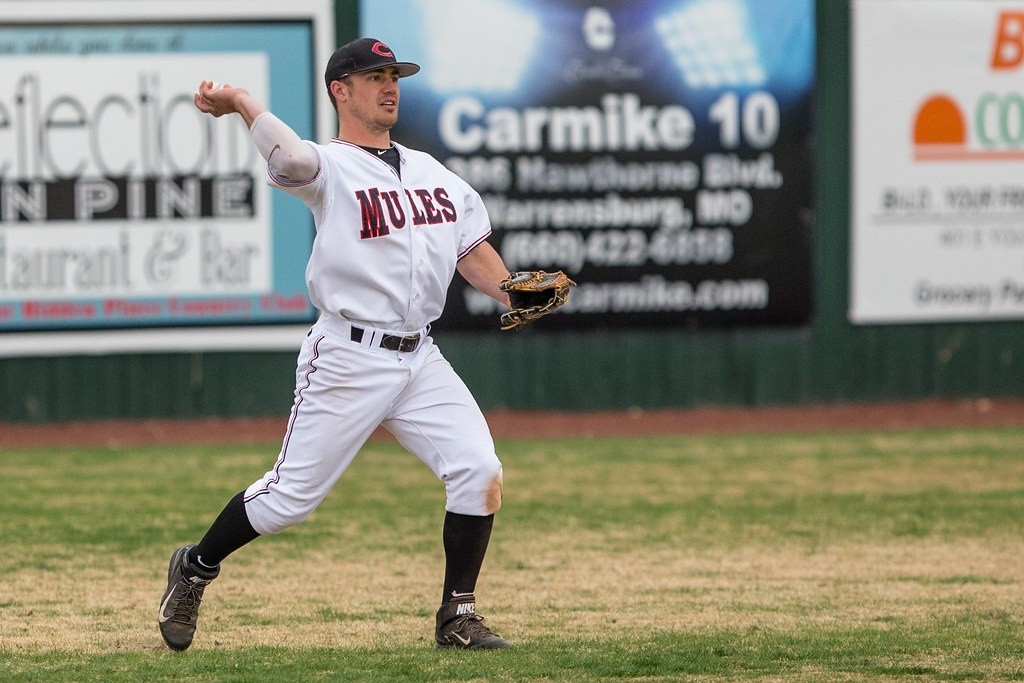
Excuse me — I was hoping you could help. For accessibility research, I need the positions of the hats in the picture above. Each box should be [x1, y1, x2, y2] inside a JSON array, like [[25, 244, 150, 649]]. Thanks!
[[324, 38, 420, 86]]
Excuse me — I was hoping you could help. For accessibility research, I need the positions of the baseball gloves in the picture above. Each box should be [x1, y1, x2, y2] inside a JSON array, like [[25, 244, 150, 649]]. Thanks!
[[498, 270, 573, 330]]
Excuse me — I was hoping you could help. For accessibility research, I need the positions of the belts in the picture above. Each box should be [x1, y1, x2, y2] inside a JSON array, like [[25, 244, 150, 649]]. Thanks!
[[351, 326, 421, 352]]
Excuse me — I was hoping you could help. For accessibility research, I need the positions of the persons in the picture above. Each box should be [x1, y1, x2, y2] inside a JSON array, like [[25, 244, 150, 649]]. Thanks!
[[159, 37, 576, 653]]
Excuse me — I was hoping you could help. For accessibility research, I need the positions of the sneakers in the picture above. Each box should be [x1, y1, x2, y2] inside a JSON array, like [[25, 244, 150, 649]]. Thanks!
[[435, 596, 505, 648], [158, 544, 221, 651]]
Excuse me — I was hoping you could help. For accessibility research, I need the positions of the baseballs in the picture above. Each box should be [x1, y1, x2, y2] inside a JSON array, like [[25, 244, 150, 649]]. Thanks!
[[207, 81, 225, 92]]
